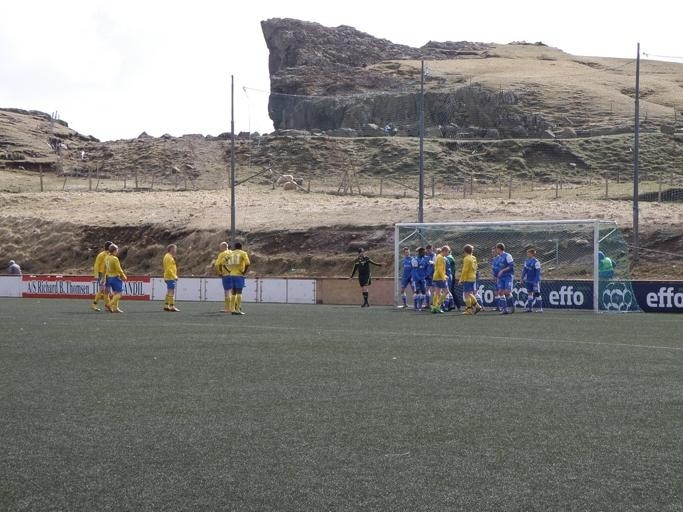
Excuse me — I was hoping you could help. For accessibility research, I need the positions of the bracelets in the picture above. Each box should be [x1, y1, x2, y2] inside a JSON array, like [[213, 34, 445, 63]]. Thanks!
[[219, 271, 222, 274]]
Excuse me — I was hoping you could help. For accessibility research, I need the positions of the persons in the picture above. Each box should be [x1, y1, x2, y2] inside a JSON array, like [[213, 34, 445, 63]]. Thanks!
[[99, 244, 127, 313], [90, 241, 112, 311], [7, 260, 21, 274], [400, 243, 515, 315], [348, 248, 385, 306], [162, 244, 180, 311], [520, 248, 543, 313], [214, 241, 241, 312], [222, 243, 250, 314], [591, 251, 616, 278]]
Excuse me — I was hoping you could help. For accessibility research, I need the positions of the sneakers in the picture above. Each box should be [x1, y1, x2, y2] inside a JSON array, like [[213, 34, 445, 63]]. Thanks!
[[402, 305, 408, 309], [220, 308, 246, 314], [104, 303, 124, 313], [163, 306, 180, 312], [361, 303, 369, 307], [412, 306, 544, 315], [90, 304, 101, 311]]
